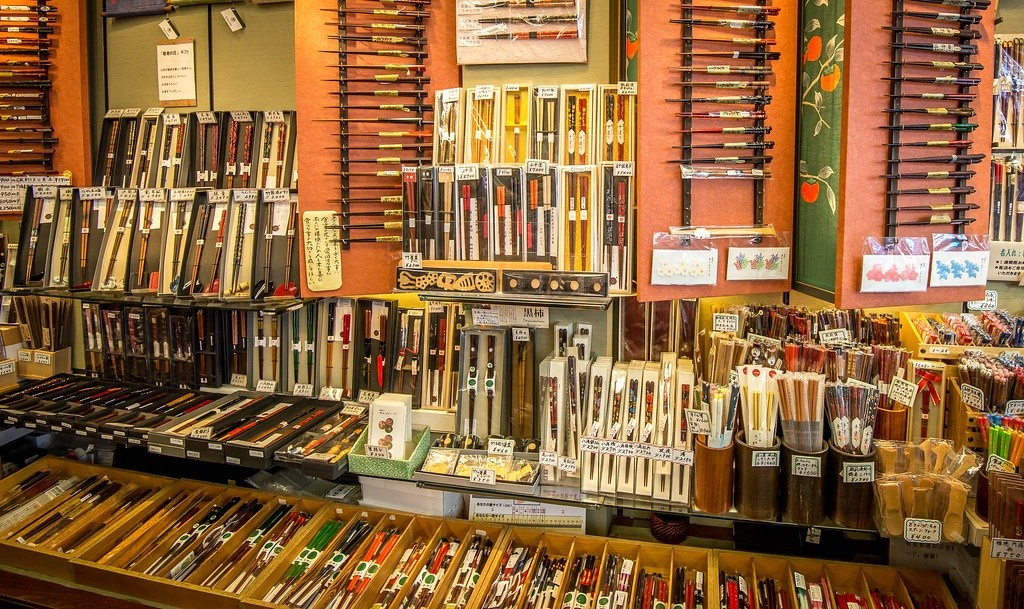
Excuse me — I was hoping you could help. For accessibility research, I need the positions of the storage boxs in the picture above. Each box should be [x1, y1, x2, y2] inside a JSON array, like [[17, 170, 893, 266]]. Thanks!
[[2, 35, 1024, 609]]
